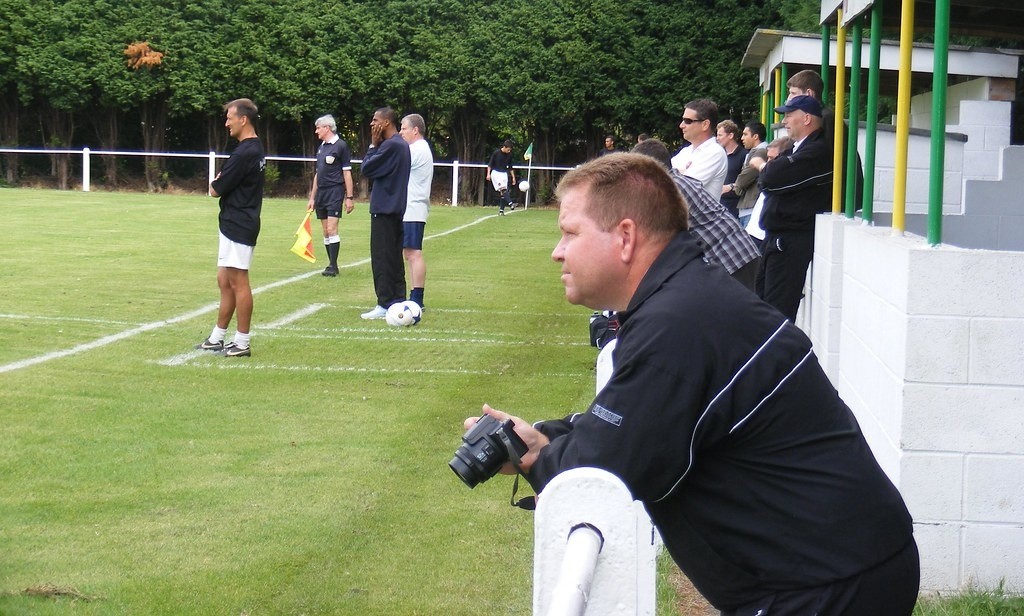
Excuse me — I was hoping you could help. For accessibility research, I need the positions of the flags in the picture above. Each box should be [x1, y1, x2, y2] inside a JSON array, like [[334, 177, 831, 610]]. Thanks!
[[291, 218, 316, 264]]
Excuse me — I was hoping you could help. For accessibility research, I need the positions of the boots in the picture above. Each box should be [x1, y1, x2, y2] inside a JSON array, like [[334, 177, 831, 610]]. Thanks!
[[322, 235, 341, 275]]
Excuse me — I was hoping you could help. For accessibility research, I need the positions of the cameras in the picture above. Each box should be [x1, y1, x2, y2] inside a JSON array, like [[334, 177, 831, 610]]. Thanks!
[[448, 413, 529, 489]]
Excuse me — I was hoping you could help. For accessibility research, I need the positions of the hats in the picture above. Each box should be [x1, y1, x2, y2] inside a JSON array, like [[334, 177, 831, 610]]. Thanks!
[[773, 95, 823, 117]]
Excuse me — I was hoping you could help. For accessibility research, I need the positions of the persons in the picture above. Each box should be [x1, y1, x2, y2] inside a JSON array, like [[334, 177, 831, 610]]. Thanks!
[[463, 153, 921, 616], [306, 113, 353, 277], [486, 141, 517, 214], [361, 108, 436, 320], [195, 98, 266, 357], [601, 69, 863, 324]]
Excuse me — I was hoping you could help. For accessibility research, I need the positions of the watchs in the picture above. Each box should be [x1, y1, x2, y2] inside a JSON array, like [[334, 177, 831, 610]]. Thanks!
[[347, 196, 353, 200], [369, 144, 377, 148]]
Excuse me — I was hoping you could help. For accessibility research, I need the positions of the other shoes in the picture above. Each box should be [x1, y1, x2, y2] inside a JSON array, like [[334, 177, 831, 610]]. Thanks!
[[511, 206, 514, 210], [499, 212, 504, 215]]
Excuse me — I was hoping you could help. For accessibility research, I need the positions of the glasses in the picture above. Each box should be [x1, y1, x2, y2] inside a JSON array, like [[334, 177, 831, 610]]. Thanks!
[[681, 118, 704, 124]]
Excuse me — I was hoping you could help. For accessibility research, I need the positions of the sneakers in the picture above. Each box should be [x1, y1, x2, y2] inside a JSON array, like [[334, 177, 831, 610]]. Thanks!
[[214, 342, 252, 356], [360, 305, 387, 320], [192, 335, 224, 351]]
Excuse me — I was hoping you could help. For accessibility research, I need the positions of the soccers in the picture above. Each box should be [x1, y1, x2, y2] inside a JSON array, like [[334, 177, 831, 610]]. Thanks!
[[386, 302, 413, 327], [402, 300, 423, 325], [518, 180, 530, 192]]
